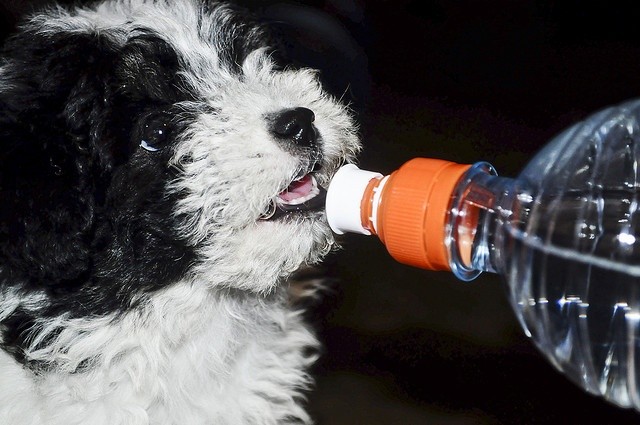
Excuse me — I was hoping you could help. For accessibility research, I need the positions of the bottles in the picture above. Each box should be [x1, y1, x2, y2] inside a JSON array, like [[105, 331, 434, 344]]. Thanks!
[[325, 97, 640, 411]]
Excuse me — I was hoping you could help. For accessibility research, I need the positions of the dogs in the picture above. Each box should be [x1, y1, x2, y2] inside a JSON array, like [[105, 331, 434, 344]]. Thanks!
[[0, 0, 362, 425]]
